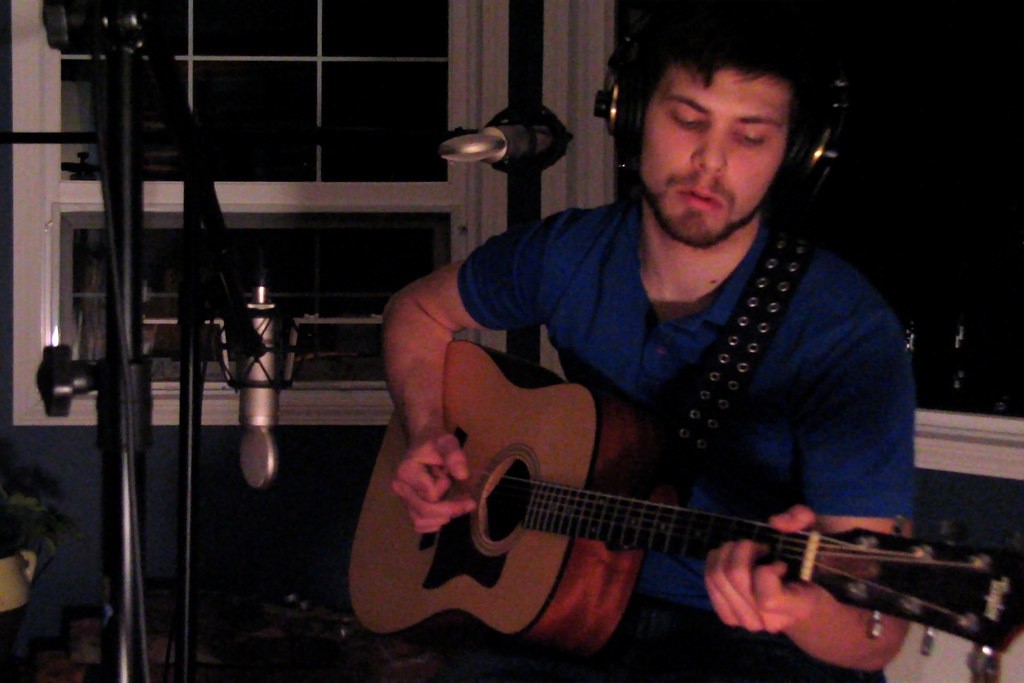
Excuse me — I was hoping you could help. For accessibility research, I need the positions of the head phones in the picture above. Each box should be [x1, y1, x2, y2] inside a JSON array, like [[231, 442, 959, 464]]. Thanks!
[[591, 38, 851, 180]]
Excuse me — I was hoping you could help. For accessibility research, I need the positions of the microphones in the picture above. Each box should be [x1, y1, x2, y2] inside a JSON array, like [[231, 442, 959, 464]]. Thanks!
[[238, 275, 278, 487], [439, 123, 554, 162]]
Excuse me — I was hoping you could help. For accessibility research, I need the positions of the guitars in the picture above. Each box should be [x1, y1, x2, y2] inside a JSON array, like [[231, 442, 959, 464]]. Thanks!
[[347, 337, 1024, 666]]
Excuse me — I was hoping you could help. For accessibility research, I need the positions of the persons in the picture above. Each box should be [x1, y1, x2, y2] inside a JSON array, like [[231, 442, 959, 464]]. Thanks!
[[381, 0, 914, 683]]
